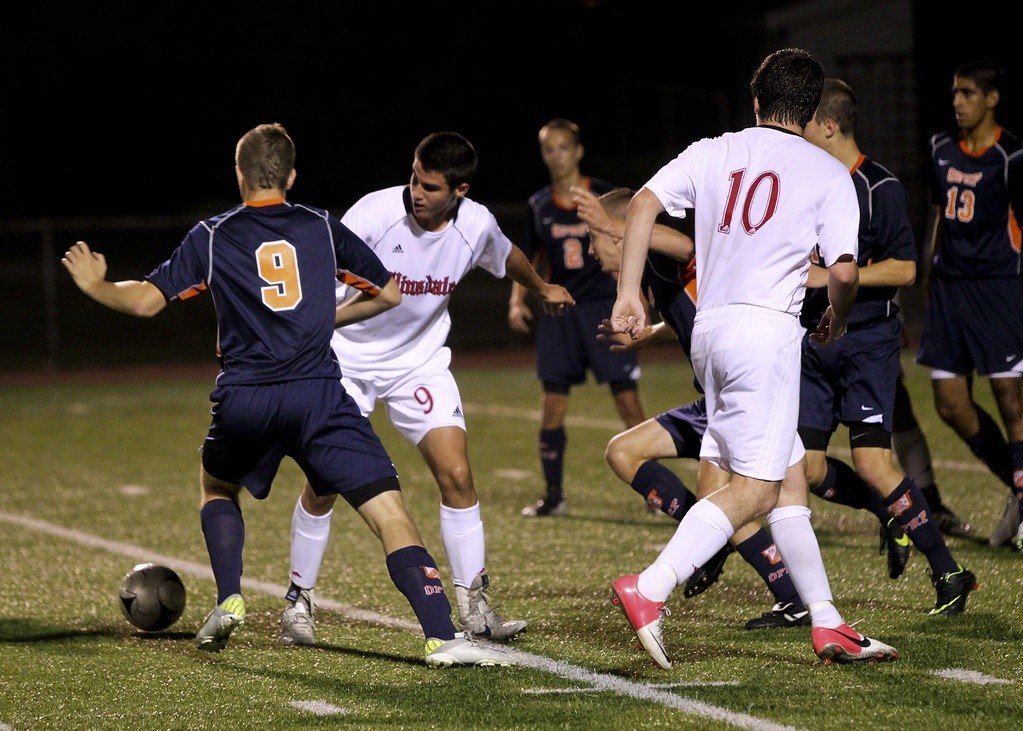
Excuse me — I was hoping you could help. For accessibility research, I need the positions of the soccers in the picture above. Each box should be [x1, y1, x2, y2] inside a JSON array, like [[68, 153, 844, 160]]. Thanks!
[[117, 562, 186, 632]]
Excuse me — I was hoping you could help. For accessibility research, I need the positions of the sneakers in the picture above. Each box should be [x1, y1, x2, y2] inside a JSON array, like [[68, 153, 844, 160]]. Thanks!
[[283, 581, 317, 645], [926, 565, 978, 616], [812, 625, 897, 665], [744, 601, 811, 629], [684, 541, 736, 599], [424, 631, 527, 666], [988, 468, 1023, 548], [878, 508, 913, 580], [196, 594, 248, 653], [455, 571, 529, 640], [928, 503, 969, 537], [610, 570, 674, 671], [521, 499, 568, 517]]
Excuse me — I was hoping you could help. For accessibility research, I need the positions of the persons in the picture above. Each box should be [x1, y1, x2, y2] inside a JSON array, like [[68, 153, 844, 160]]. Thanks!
[[282, 129, 528, 643], [798, 80, 981, 615], [608, 47, 899, 672], [61, 120, 516, 667], [911, 61, 1022, 547], [507, 119, 646, 514], [569, 185, 813, 630]]
